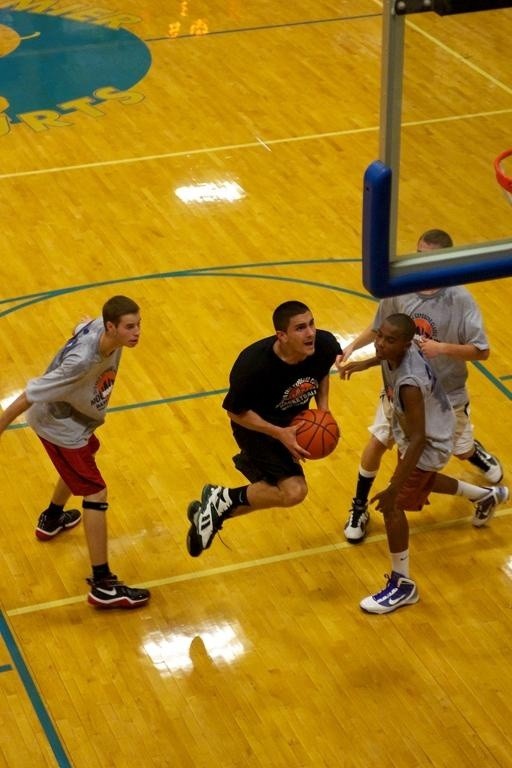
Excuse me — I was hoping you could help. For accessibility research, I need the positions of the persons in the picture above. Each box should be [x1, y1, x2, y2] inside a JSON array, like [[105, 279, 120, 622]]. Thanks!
[[335, 228, 506, 543], [341, 312, 509, 616], [0, 293, 151, 611], [185, 301, 344, 557]]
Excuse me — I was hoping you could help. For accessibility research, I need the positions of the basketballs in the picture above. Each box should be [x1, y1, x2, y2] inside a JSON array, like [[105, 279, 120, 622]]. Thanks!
[[289, 408, 343, 459]]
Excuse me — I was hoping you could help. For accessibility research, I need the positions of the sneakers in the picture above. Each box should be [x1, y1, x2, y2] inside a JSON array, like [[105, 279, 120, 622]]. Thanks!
[[35, 509, 81, 540], [187, 484, 233, 556], [360, 570, 420, 613], [467, 439, 503, 485], [468, 487, 509, 528], [86, 575, 150, 608], [344, 498, 369, 542]]
